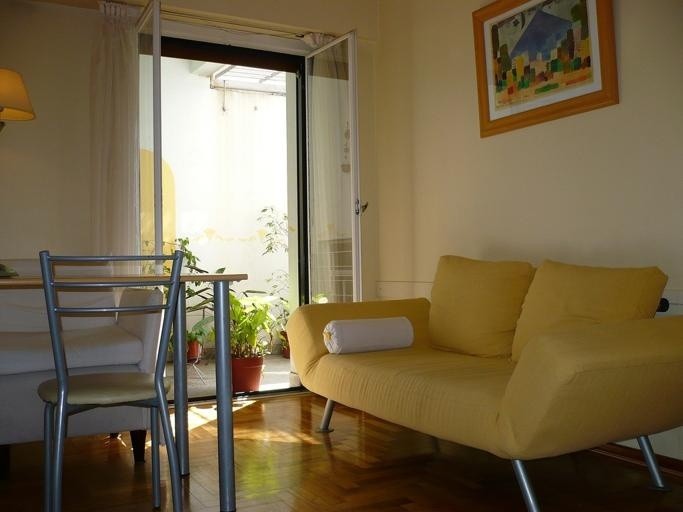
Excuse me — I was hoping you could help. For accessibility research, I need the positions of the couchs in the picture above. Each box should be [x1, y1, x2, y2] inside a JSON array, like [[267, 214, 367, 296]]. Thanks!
[[1, 254, 165, 463], [286, 252, 682, 506]]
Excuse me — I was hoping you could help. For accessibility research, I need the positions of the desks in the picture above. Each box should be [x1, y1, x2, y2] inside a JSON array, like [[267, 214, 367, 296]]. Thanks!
[[2, 271, 250, 493]]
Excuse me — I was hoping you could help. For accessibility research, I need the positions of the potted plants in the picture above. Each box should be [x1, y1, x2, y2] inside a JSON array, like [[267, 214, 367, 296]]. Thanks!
[[187, 314, 213, 366], [221, 294, 277, 396]]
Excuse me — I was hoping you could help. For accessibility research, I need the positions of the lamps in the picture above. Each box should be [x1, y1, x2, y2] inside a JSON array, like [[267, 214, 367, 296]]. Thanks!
[[1, 68, 39, 150]]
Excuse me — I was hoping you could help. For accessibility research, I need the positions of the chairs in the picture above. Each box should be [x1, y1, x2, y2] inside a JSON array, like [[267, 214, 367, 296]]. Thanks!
[[37, 250, 183, 512]]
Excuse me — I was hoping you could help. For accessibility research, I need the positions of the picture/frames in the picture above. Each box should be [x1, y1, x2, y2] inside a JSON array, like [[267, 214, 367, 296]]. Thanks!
[[469, 1, 625, 142]]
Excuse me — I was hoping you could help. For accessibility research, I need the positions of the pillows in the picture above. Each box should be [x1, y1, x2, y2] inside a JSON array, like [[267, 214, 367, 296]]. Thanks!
[[321, 314, 418, 358]]
